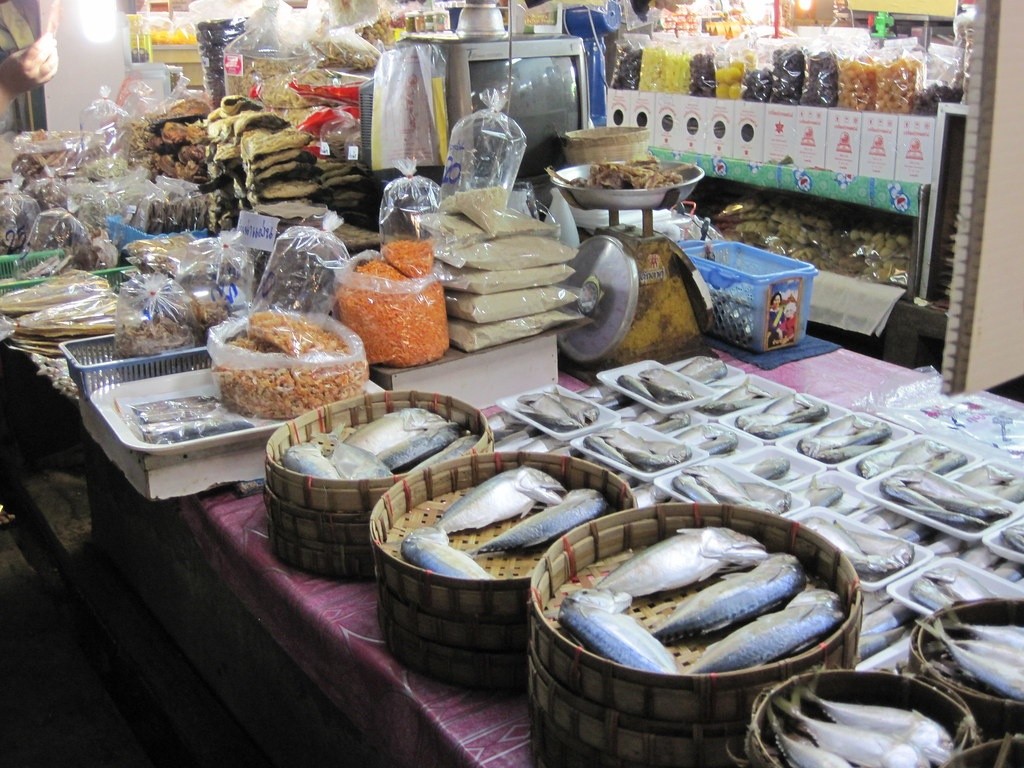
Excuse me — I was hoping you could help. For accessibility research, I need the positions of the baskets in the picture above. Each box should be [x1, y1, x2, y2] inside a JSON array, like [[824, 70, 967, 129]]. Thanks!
[[682, 240, 818, 353], [561, 126, 650, 167]]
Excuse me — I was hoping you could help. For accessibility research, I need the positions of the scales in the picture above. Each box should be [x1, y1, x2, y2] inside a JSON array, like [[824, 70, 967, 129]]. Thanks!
[[549, 161, 711, 370]]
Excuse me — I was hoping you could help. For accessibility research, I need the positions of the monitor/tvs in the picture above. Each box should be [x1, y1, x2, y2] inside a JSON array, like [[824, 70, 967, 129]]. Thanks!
[[359, 32, 591, 214]]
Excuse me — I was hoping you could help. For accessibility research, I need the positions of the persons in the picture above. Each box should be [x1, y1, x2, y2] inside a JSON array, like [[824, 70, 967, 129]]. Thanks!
[[0, 32, 59, 528], [769, 292, 796, 344]]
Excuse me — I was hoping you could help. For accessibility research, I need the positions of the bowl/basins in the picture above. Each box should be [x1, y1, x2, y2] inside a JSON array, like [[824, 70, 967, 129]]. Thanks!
[[551, 160, 705, 209]]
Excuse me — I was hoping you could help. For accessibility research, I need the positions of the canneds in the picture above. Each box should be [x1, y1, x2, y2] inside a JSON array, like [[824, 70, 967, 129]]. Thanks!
[[405, 9, 451, 32]]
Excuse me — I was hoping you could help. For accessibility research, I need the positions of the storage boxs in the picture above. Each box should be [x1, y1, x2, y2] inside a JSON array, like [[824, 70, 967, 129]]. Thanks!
[[682, 240, 819, 354], [605, 85, 934, 185]]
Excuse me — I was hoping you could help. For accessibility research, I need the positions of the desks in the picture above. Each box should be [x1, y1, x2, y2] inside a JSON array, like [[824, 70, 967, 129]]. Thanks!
[[0, 335, 1024, 768]]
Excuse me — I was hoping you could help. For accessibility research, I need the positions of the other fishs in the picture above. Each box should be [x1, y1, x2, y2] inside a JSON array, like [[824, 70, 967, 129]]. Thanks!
[[278, 354, 1024, 768]]
